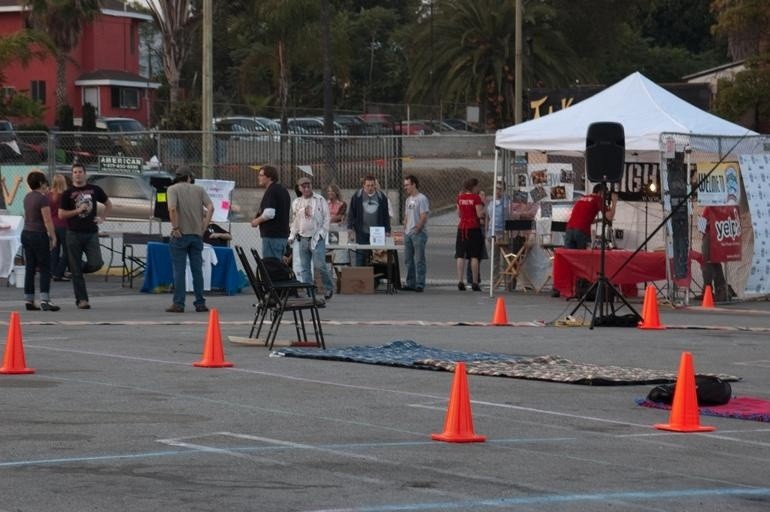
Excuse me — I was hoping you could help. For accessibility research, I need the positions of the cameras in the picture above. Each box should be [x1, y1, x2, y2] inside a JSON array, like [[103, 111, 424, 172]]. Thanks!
[[605, 190, 613, 202]]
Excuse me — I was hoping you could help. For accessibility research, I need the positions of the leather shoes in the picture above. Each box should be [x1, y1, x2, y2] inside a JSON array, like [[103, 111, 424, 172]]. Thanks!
[[324, 289, 332, 299], [458, 282, 466, 291], [78, 299, 91, 309], [472, 283, 481, 291], [196, 303, 208, 311], [166, 304, 184, 312], [53, 276, 71, 281]]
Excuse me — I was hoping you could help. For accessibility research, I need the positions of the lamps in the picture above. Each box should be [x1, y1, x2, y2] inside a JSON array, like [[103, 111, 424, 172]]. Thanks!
[[644, 182, 656, 194]]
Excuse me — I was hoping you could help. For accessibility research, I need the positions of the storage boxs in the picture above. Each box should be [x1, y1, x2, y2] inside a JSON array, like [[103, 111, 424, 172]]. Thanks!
[[333, 265, 384, 294]]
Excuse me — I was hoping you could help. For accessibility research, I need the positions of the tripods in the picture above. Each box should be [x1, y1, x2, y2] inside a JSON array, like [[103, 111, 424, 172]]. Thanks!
[[566, 184, 643, 329], [606, 205, 618, 249]]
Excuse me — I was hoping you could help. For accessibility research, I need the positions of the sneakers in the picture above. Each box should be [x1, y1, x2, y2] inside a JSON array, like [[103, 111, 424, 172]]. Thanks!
[[398, 286, 423, 293]]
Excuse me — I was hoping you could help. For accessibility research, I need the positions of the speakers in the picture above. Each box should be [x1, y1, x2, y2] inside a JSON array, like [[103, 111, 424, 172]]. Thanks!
[[586, 122, 625, 183]]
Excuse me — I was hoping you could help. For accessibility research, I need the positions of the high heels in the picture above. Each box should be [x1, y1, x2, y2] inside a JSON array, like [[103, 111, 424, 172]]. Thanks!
[[25, 302, 41, 310], [41, 299, 60, 311]]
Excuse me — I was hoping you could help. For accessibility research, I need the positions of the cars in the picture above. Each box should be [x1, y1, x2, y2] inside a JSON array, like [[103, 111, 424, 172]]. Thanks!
[[0, 120, 23, 159], [63, 169, 163, 221]]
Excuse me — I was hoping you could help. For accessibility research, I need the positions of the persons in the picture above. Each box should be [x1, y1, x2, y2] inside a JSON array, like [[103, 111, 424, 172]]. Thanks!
[[0, 163, 112, 311], [485, 181, 511, 283], [690, 169, 700, 202], [532, 170, 547, 184], [550, 183, 619, 297], [203, 215, 233, 247], [165, 166, 214, 313], [467, 188, 488, 284], [454, 178, 486, 291], [252, 165, 431, 307], [519, 175, 526, 186]]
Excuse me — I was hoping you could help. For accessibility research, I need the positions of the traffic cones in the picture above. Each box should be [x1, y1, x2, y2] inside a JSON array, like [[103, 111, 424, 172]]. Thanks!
[[0, 308, 38, 376], [655, 348, 715, 435], [427, 358, 491, 445], [191, 307, 237, 370], [490, 295, 512, 327], [635, 283, 668, 330], [700, 284, 716, 309]]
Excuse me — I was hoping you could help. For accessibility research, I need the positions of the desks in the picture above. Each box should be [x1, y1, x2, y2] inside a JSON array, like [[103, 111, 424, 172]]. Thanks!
[[325, 243, 404, 294], [98, 231, 142, 281], [0, 215, 23, 287], [552, 248, 665, 297], [140, 240, 239, 296]]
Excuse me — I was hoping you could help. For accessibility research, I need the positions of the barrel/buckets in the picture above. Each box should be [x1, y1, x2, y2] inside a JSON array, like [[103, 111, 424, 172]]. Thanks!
[[13, 265, 26, 289]]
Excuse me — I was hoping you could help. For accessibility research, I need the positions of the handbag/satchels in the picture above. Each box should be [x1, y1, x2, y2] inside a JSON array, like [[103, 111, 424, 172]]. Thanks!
[[648, 373, 732, 407]]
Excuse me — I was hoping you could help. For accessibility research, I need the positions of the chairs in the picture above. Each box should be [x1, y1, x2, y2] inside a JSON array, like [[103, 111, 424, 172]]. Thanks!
[[250, 247, 325, 351], [538, 220, 568, 293], [122, 232, 163, 288], [233, 244, 308, 343], [493, 219, 536, 294]]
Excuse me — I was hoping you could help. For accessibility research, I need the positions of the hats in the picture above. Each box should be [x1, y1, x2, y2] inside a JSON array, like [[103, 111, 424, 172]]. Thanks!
[[176, 166, 197, 178], [298, 177, 311, 185]]
